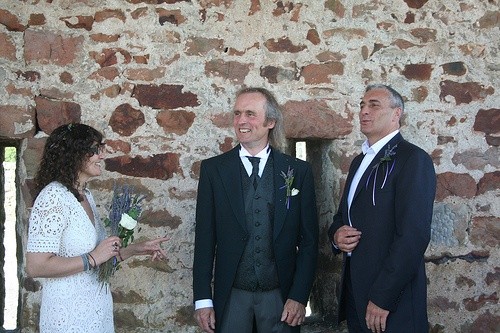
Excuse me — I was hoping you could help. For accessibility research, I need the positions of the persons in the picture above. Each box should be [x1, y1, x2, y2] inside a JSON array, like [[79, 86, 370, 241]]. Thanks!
[[25, 122, 172, 333], [328, 84, 437, 333], [192, 87, 320, 333]]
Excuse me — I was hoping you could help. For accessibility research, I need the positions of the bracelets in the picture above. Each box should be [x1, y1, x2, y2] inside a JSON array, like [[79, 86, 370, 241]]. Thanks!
[[118, 248, 124, 261], [80, 253, 96, 271]]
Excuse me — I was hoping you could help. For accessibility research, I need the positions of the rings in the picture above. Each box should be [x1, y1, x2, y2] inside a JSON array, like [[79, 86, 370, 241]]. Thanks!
[[113, 241, 118, 249]]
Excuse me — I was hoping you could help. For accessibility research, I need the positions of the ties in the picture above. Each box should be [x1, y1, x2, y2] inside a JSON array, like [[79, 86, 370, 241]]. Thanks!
[[245, 156, 261, 192]]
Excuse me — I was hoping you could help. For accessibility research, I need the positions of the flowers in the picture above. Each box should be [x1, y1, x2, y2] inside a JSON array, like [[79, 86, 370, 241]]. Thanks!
[[279, 165, 295, 209], [89, 184, 147, 295], [366, 144, 399, 206]]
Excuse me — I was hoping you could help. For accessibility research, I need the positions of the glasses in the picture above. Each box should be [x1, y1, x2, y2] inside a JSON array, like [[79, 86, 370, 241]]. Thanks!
[[89, 146, 105, 155]]
